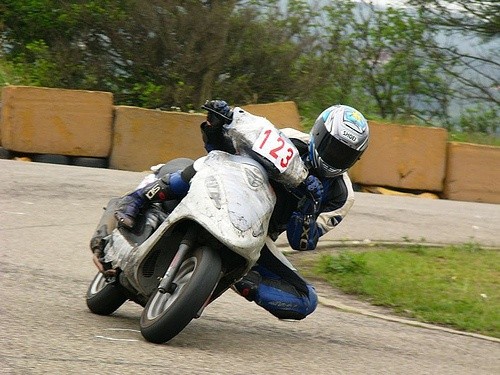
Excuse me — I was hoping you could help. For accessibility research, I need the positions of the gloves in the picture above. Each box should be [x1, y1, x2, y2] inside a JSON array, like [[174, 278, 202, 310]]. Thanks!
[[205, 100, 234, 131], [297, 175, 323, 217]]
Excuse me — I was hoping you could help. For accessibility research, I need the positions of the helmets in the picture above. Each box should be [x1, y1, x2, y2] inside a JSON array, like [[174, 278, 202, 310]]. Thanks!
[[308, 105, 369, 177]]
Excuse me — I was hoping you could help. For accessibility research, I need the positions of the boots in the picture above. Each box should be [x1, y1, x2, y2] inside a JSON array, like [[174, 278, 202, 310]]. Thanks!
[[230, 271, 262, 302], [114, 172, 172, 237]]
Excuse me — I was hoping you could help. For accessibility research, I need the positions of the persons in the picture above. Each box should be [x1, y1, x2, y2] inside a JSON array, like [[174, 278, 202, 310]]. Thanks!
[[113, 100, 369, 324]]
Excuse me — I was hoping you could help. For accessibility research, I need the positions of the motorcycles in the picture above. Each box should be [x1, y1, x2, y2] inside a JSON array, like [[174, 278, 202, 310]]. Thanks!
[[86, 99, 317, 347]]
